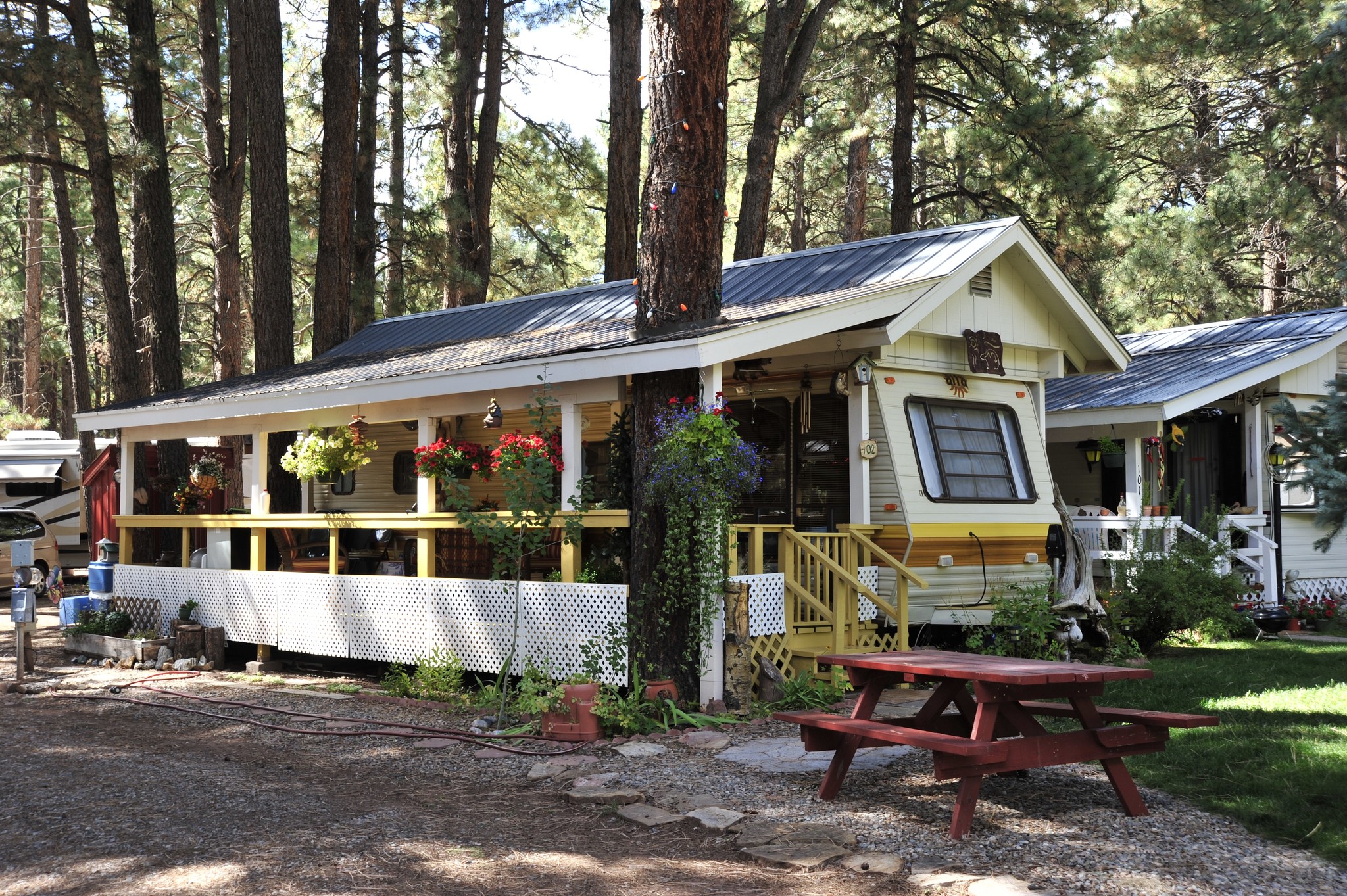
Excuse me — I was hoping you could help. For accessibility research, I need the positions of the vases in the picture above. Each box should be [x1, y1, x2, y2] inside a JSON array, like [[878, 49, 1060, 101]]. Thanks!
[[1151, 506, 1161, 516], [445, 463, 473, 479], [316, 467, 342, 482], [190, 474, 218, 489], [1287, 619, 1330, 631]]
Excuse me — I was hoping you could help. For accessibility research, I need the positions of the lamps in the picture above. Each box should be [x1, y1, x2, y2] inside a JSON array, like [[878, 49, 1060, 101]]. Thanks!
[[732, 383, 750, 396], [1075, 439, 1102, 463], [1023, 552, 1038, 563], [937, 555, 953, 566], [1007, 623, 1130, 642], [1267, 442, 1285, 466]]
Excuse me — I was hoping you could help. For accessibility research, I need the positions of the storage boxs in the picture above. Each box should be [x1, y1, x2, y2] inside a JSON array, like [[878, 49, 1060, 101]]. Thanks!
[[60, 561, 118, 625]]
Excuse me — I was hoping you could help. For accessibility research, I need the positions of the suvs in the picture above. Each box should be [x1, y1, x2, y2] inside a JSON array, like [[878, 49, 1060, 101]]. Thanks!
[[0, 505, 62, 599]]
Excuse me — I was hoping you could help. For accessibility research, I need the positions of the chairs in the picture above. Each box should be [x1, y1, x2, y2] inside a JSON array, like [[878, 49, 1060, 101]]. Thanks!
[[1066, 505, 1127, 588], [269, 509, 561, 581]]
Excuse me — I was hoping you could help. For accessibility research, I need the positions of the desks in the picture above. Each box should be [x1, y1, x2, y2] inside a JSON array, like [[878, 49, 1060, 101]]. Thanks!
[[394, 536, 417, 561], [816, 651, 1153, 839]]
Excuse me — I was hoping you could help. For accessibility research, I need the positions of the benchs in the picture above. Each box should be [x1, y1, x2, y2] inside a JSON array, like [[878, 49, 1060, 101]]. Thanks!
[[772, 699, 1220, 757]]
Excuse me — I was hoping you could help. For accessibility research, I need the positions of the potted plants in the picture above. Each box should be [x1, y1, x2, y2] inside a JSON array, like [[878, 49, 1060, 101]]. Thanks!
[[1097, 436, 1126, 468], [1142, 478, 1169, 516], [541, 673, 678, 740], [65, 600, 199, 663]]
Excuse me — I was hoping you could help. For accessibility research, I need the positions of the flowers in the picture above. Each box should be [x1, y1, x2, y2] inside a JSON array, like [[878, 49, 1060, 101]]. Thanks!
[[170, 423, 588, 515], [622, 392, 771, 676], [1277, 596, 1342, 621]]
